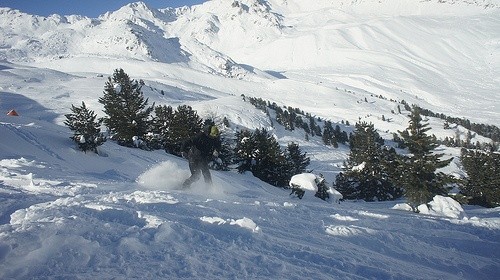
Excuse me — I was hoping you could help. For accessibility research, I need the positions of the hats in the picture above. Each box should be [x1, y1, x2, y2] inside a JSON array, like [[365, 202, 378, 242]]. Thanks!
[[209, 126, 219, 136]]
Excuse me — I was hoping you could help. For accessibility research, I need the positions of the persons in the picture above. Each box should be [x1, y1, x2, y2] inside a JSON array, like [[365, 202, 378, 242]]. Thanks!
[[180, 124, 221, 191]]
